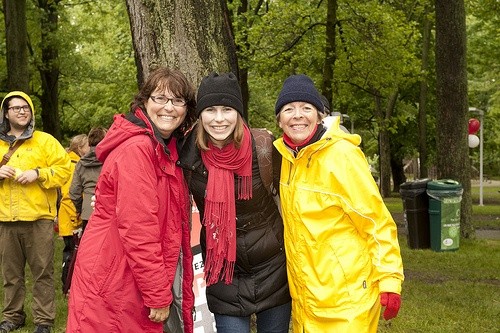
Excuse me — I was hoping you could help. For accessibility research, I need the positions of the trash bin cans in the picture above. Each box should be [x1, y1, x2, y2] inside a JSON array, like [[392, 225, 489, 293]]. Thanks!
[[426, 178, 464, 253], [399, 178, 431, 251]]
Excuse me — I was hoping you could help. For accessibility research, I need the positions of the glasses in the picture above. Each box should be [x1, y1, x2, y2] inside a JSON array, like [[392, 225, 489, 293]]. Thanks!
[[147, 93, 187, 107], [4, 104, 32, 113]]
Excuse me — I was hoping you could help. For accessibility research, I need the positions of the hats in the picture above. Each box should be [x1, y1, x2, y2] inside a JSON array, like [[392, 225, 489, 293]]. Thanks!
[[196, 70, 243, 117], [275, 75, 325, 115]]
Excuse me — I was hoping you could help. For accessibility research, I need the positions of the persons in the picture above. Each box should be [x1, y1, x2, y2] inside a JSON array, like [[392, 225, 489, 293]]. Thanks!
[[91, 74, 291, 333], [54, 127, 112, 296], [259, 75, 404, 333], [0, 91, 72, 333], [67, 70, 194, 333]]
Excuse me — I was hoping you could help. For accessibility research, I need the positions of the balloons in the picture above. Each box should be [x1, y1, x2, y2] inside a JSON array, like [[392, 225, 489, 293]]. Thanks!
[[468, 118, 480, 148]]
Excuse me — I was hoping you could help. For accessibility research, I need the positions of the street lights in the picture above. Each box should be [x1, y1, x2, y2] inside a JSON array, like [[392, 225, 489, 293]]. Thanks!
[[468, 107, 484, 206], [342, 114, 354, 134]]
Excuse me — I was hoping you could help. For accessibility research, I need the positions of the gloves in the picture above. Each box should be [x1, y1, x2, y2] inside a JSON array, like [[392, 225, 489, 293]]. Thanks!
[[380, 291, 402, 321]]
